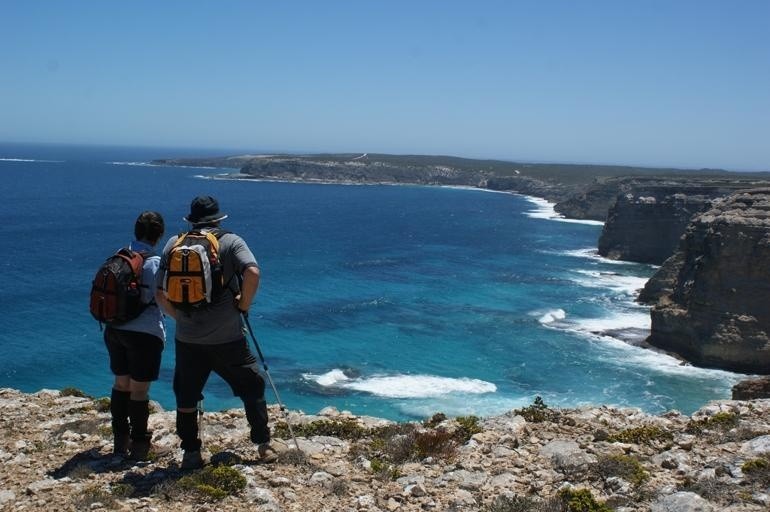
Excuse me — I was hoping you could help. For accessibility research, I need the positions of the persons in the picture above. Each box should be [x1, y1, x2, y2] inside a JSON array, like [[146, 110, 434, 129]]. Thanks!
[[102, 209, 166, 460], [153, 194, 288, 472]]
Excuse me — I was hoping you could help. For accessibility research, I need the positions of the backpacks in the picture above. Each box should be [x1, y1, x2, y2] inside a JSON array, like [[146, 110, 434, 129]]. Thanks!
[[88, 242, 160, 326], [166, 228, 238, 311]]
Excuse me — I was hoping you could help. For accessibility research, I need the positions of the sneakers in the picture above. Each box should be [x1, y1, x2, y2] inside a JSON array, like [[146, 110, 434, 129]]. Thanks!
[[257, 437, 289, 463], [181, 447, 214, 467]]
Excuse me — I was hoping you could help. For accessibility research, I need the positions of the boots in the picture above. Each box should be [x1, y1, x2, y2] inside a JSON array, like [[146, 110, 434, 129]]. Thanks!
[[115, 428, 171, 460]]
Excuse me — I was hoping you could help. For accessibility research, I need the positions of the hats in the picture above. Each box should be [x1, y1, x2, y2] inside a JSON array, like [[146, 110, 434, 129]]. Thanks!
[[182, 196, 229, 225]]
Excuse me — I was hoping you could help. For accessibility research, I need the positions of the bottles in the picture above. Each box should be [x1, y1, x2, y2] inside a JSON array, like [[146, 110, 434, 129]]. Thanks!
[[128, 280, 139, 298]]
